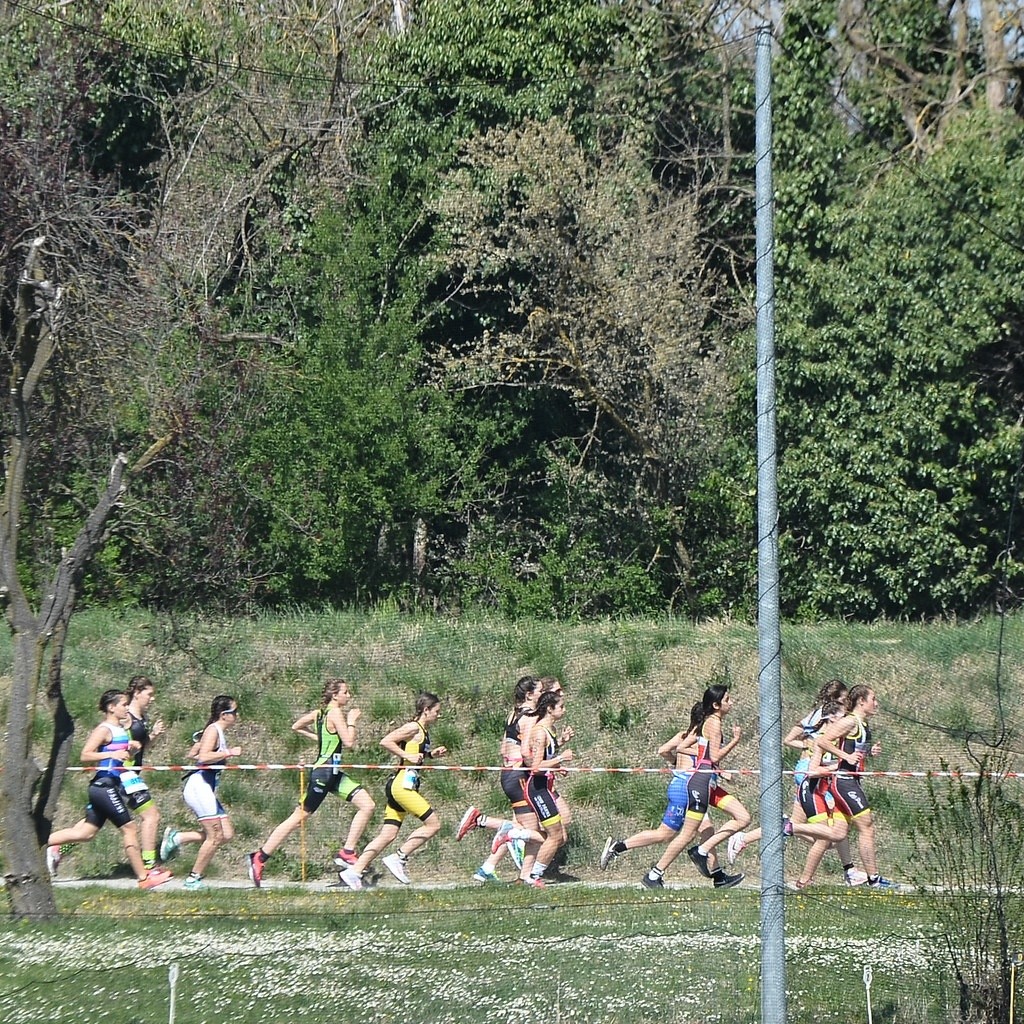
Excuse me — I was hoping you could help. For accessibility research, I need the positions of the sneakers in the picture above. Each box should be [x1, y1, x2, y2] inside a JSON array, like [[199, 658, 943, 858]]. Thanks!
[[600, 836, 620, 871], [844, 867, 868, 887], [184, 872, 206, 888], [456, 806, 480, 842], [47, 845, 61, 877], [714, 873, 746, 888], [868, 875, 901, 887], [382, 854, 410, 884], [334, 849, 370, 874], [473, 867, 500, 882], [514, 877, 545, 889], [640, 874, 664, 889], [138, 865, 174, 889], [529, 873, 557, 883], [687, 846, 710, 878], [783, 813, 790, 836], [795, 879, 816, 889], [728, 832, 746, 865], [339, 868, 363, 891], [160, 826, 182, 861], [491, 820, 513, 854], [506, 839, 525, 870], [247, 851, 265, 887]]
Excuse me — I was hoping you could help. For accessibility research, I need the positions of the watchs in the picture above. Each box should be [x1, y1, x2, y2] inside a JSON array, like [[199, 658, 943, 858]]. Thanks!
[[558, 755, 564, 763]]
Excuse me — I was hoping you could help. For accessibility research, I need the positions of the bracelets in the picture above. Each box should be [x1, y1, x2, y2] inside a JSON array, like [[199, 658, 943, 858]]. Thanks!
[[347, 724, 356, 728], [223, 748, 232, 757], [672, 758, 677, 765], [428, 751, 433, 759]]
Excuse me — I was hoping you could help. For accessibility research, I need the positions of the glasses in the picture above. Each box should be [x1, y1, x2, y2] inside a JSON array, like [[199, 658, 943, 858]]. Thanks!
[[554, 688, 563, 696], [223, 706, 238, 714]]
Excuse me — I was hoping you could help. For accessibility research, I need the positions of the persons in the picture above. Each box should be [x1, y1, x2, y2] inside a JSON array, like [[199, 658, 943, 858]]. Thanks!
[[46, 675, 174, 877], [246, 678, 375, 888], [600, 702, 746, 890], [640, 683, 751, 891], [455, 675, 574, 889], [337, 692, 447, 890], [727, 679, 901, 890], [160, 695, 241, 890], [48, 689, 175, 893]]
